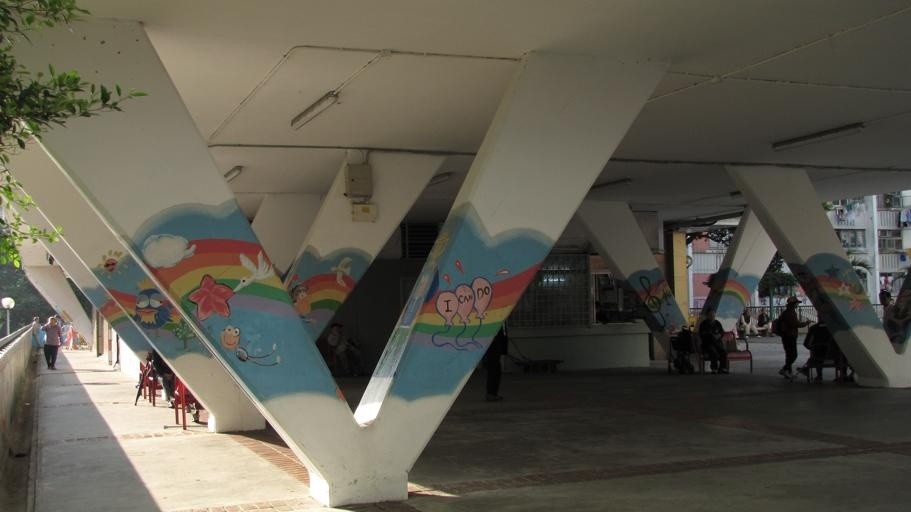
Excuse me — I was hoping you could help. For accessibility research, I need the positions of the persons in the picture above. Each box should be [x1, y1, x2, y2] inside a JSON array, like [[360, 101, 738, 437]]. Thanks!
[[699, 308, 727, 374], [879, 291, 896, 322], [740, 308, 776, 339], [40, 317, 62, 370], [797, 314, 848, 382], [327, 321, 378, 379], [33, 314, 82, 351], [148, 349, 175, 408], [778, 296, 811, 378], [481, 325, 503, 401]]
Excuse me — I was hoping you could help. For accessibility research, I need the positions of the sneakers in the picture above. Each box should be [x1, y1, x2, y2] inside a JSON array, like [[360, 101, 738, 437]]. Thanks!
[[778, 369, 798, 380]]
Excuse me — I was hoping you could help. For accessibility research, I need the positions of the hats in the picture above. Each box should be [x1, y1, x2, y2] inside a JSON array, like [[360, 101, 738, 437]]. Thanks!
[[786, 296, 802, 305]]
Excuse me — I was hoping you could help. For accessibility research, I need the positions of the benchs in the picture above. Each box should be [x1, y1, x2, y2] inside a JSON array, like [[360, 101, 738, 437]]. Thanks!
[[669, 332, 753, 375]]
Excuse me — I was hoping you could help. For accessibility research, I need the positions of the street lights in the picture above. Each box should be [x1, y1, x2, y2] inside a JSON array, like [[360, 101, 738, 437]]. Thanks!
[[1, 297, 16, 336]]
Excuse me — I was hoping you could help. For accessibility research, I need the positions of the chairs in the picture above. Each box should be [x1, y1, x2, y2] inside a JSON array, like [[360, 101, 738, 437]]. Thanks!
[[144, 360, 205, 430]]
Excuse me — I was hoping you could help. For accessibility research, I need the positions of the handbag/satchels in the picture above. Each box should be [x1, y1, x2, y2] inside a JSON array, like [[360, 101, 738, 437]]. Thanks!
[[771, 316, 785, 337]]
